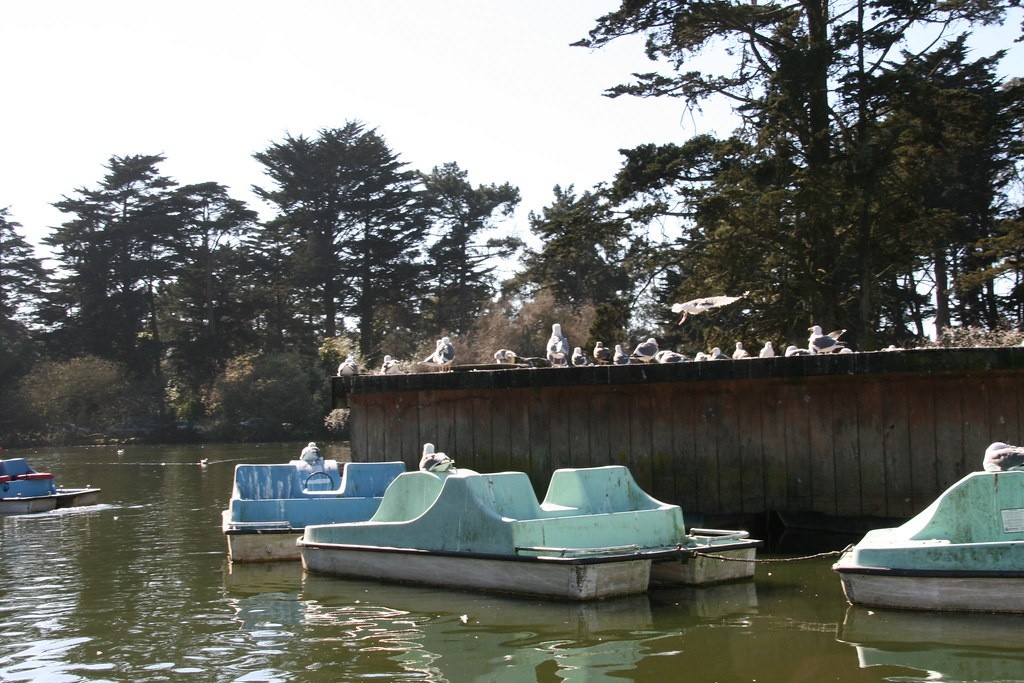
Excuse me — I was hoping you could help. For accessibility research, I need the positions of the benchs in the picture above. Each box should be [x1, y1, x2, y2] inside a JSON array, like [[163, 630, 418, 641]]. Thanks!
[[226, 457, 686, 559]]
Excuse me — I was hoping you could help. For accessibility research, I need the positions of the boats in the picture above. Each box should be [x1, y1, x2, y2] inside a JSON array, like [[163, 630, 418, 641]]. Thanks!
[[222, 441, 406, 563], [296, 466, 764, 601], [0, 457, 101, 515], [831, 441, 1024, 613]]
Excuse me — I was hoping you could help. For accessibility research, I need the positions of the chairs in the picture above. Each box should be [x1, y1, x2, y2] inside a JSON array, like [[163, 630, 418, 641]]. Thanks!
[[0, 458, 56, 498]]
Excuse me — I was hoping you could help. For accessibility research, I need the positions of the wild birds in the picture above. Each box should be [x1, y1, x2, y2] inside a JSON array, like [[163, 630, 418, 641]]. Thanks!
[[335, 358, 359, 377], [494, 349, 518, 363], [299, 442, 322, 461], [117, 448, 126, 453], [201, 458, 209, 464], [423, 337, 457, 372], [546, 296, 848, 365], [418, 442, 454, 472], [982, 442, 1024, 472], [380, 355, 405, 375]]
